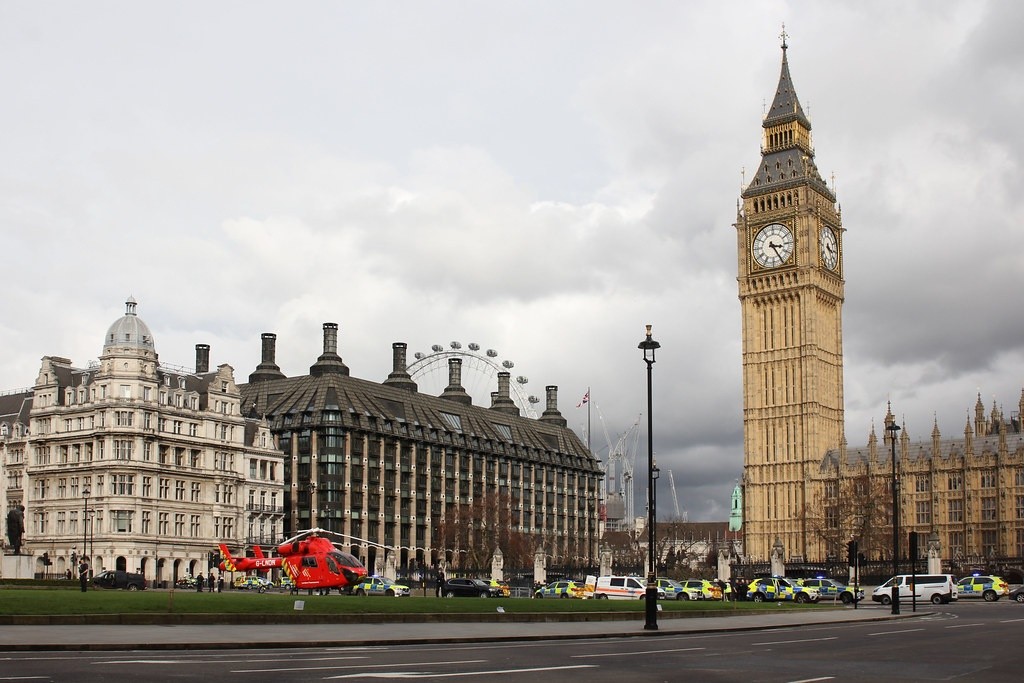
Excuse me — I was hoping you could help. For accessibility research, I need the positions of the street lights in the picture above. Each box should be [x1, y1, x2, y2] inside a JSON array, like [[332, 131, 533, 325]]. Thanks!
[[83, 489, 91, 556], [638, 323, 661, 630], [71, 552, 77, 567], [886, 419, 902, 615]]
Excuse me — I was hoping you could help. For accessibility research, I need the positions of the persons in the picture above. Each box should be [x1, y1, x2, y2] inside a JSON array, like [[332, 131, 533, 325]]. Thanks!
[[531, 580, 547, 598], [717, 578, 748, 602], [79, 557, 88, 592], [66, 569, 72, 580], [196, 571, 224, 592], [435, 568, 446, 597]]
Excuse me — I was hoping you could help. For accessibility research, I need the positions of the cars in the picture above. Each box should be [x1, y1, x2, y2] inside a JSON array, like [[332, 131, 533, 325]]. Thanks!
[[678, 580, 723, 602], [352, 575, 410, 597], [746, 577, 820, 604], [635, 577, 698, 601], [535, 581, 585, 599], [1008, 584, 1024, 603], [956, 575, 1010, 601], [801, 578, 865, 604], [441, 578, 510, 599]]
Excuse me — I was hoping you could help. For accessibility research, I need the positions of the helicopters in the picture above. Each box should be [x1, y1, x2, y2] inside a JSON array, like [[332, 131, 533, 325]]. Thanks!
[[218, 527, 396, 589]]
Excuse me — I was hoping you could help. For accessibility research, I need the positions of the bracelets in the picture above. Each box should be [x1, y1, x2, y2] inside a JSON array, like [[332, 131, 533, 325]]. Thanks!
[[82, 572, 84, 574]]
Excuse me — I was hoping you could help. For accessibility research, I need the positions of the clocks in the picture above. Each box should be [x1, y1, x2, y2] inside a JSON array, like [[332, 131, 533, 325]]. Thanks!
[[752, 224, 794, 268], [820, 227, 839, 270]]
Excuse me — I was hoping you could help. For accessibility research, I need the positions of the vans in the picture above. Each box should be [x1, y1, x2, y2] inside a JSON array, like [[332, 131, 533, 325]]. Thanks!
[[584, 575, 666, 601], [871, 574, 958, 605]]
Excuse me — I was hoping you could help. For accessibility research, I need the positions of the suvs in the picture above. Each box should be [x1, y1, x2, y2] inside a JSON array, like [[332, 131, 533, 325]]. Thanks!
[[87, 571, 148, 592], [281, 577, 295, 589], [234, 575, 275, 590]]
